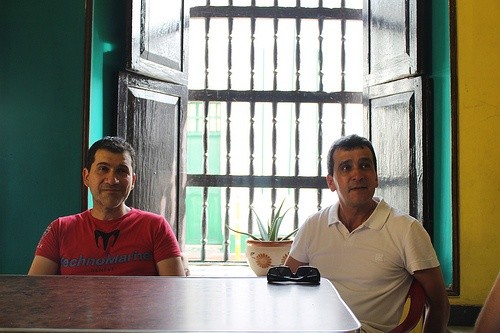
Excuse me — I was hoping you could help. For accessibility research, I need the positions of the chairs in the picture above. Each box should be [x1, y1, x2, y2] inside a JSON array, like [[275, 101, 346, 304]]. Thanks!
[[386, 275, 428, 333]]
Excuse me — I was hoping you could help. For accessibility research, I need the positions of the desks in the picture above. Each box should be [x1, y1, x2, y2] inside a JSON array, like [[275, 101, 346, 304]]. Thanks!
[[0, 273, 363, 333]]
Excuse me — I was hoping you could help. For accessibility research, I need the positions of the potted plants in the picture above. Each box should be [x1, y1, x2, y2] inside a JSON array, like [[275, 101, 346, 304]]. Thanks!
[[225, 200, 300, 276]]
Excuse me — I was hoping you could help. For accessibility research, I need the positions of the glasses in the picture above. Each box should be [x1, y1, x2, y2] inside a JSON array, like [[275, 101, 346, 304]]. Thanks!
[[266, 264, 321, 285]]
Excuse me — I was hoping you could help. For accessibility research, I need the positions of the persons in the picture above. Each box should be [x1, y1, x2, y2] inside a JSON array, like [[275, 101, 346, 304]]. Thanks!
[[280, 134, 450, 333], [473, 271, 500, 333], [28, 136, 186, 276]]
